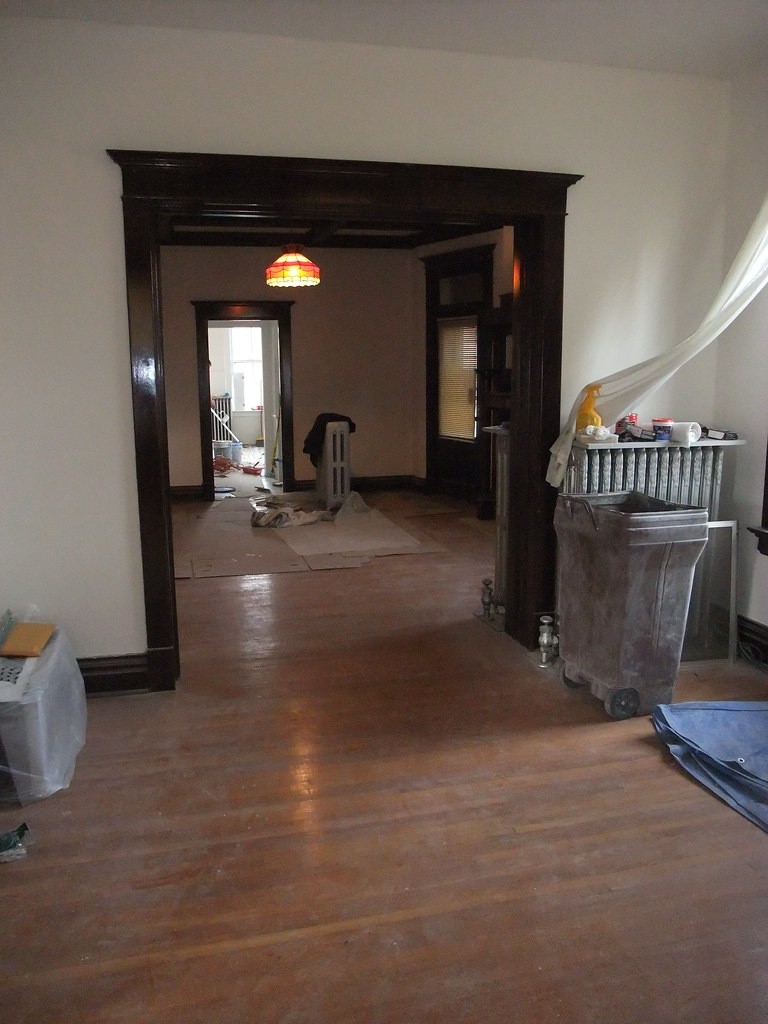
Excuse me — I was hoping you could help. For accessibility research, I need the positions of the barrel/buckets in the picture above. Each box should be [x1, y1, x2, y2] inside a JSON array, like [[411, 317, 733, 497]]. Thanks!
[[212, 441, 232, 458], [229, 441, 242, 462]]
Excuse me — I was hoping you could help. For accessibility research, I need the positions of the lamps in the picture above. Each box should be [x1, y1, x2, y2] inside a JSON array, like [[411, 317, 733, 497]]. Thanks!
[[266, 242, 320, 288]]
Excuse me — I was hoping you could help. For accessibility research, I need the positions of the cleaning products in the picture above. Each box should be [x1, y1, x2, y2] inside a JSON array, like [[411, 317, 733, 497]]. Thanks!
[[576, 384, 602, 434]]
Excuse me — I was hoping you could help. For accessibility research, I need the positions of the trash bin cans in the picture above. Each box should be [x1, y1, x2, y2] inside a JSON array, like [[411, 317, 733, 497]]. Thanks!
[[553, 484, 707, 719]]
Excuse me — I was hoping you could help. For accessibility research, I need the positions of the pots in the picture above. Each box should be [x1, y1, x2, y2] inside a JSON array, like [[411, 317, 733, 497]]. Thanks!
[[243, 462, 261, 474]]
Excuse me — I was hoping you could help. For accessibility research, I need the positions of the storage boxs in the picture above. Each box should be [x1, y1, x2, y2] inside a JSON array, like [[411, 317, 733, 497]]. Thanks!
[[576, 435, 619, 443], [627, 425, 655, 440], [708, 429, 738, 440]]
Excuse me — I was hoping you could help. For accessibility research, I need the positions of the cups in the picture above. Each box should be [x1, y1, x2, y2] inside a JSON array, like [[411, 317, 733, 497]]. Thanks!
[[652, 419, 673, 442]]
